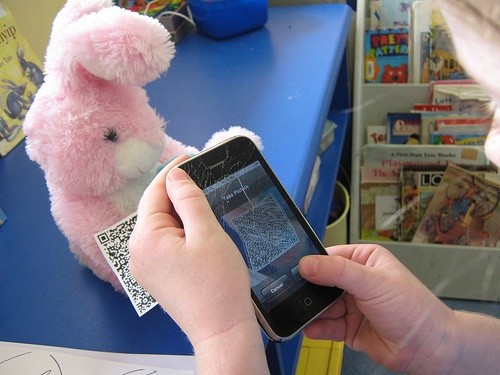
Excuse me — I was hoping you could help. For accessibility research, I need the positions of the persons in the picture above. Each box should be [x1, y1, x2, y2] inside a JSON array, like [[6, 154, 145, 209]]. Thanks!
[[128, 0, 499, 375]]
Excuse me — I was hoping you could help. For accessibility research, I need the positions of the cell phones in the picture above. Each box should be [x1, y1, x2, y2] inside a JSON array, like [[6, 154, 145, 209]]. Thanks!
[[170, 135, 346, 344]]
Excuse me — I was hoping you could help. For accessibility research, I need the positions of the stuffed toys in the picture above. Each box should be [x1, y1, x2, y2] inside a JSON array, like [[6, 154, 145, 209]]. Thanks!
[[22, 0, 263, 299]]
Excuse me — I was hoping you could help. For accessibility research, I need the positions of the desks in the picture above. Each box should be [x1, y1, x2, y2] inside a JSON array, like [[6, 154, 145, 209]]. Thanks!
[[1, 1, 351, 375]]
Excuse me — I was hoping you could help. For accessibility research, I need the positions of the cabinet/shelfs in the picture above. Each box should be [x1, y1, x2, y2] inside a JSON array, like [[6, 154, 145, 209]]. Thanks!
[[352, 1, 499, 302]]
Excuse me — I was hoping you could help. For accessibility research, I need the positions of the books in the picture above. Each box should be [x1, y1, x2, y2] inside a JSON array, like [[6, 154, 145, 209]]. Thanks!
[[361, 0, 500, 250]]
